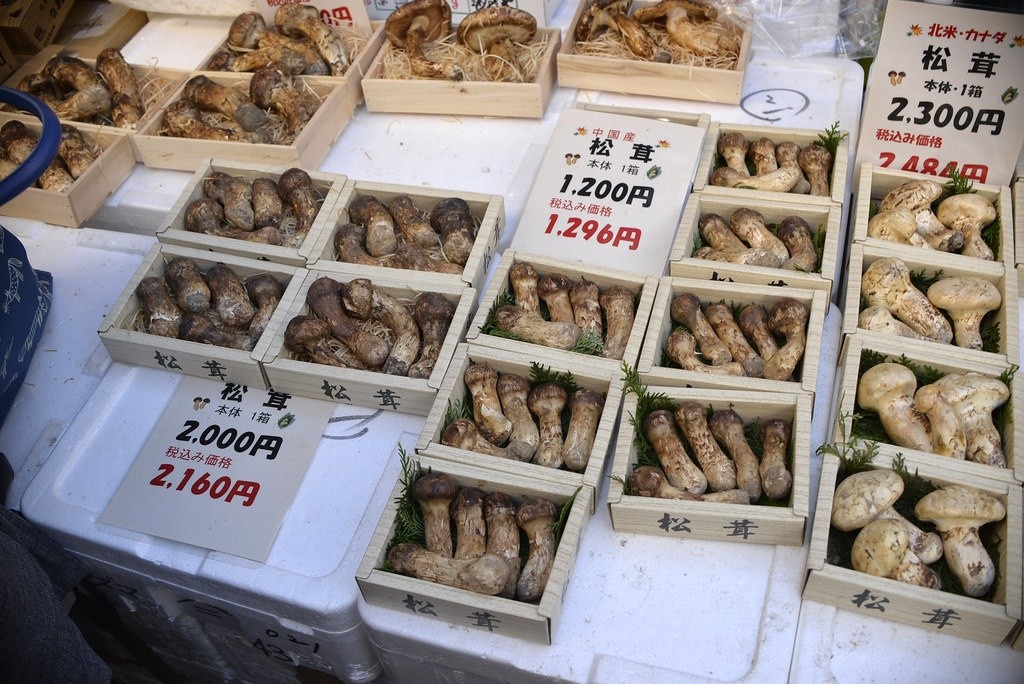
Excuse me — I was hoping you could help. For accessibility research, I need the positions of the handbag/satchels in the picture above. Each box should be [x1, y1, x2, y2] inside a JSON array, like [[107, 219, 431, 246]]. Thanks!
[[0, 87, 63, 430]]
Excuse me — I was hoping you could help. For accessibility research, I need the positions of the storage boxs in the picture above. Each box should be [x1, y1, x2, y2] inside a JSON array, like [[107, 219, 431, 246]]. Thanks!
[[788, 298, 1024, 684], [413, 342, 628, 516], [354, 454, 592, 645], [0, 214, 158, 514], [18, 364, 428, 684], [357, 300, 844, 684], [824, 338, 1024, 487], [800, 444, 1024, 648], [0, 0, 1024, 417], [605, 384, 813, 548]]
